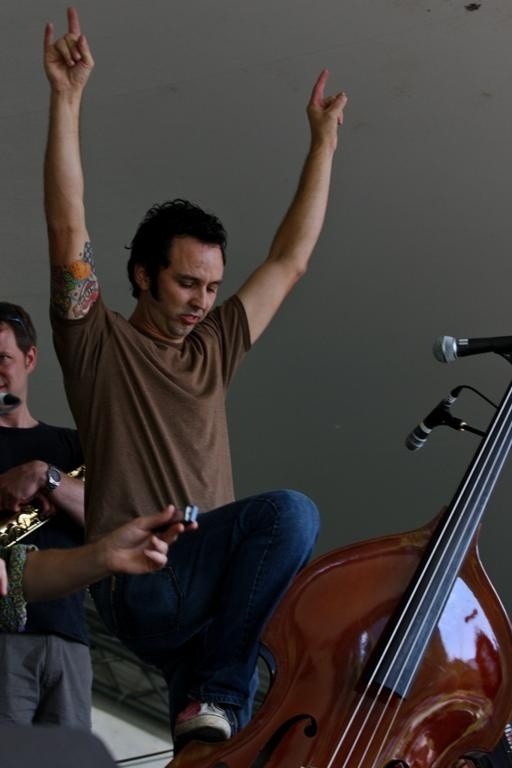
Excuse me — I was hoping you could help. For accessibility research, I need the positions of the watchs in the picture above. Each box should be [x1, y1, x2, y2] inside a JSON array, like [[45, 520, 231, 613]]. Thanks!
[[42, 466, 61, 496]]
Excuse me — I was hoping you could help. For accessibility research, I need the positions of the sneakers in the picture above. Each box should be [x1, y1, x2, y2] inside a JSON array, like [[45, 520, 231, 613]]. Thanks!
[[173, 697, 233, 749]]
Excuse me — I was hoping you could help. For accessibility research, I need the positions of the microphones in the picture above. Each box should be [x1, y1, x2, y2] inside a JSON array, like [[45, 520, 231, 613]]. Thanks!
[[432, 335, 512, 362], [0, 392, 20, 405], [405, 385, 462, 453]]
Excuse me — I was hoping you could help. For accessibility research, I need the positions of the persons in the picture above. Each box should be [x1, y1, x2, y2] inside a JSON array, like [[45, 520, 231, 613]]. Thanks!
[[1, 504, 197, 607], [42, 5, 350, 751], [0, 301, 92, 736]]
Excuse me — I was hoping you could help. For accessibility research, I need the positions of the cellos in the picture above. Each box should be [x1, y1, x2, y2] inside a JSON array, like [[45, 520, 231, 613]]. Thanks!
[[171, 373, 511, 768]]
